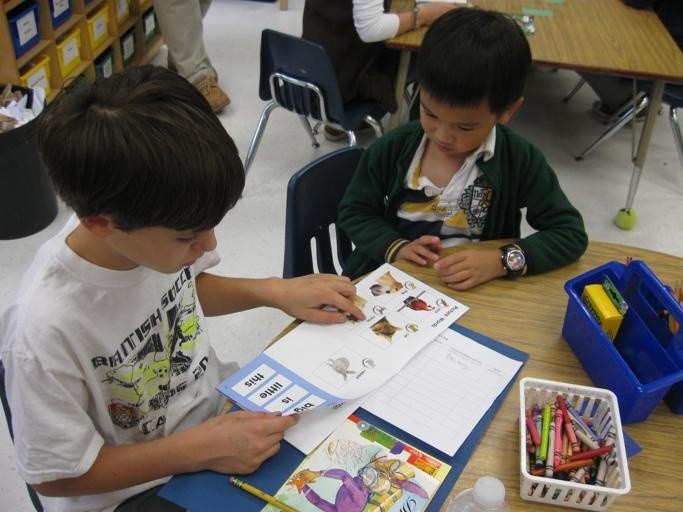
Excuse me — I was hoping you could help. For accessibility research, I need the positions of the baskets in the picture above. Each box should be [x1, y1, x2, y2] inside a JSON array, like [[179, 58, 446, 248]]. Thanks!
[[520, 376, 631, 511]]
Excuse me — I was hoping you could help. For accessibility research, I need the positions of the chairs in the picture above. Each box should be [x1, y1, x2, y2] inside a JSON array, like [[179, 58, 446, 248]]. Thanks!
[[0, 356, 45, 512]]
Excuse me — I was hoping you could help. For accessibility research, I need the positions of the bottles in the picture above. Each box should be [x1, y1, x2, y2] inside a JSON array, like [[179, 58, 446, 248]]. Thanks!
[[445, 476, 510, 512]]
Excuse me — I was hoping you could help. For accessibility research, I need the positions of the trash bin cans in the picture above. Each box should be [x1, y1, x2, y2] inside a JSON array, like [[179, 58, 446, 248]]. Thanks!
[[1, 83, 58, 239]]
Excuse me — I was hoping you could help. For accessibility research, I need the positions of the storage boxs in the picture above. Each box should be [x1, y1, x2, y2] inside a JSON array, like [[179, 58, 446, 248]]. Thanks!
[[8, 0, 158, 100]]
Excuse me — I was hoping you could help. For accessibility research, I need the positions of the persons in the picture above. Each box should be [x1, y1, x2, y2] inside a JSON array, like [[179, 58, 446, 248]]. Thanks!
[[0, 62, 366, 512], [334, 8, 588, 313], [296, 0, 461, 141], [153, 2, 231, 116], [580, 0, 683, 125]]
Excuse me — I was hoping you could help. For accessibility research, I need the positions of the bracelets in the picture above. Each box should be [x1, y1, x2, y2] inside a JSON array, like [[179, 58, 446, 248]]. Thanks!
[[410, 8, 421, 30]]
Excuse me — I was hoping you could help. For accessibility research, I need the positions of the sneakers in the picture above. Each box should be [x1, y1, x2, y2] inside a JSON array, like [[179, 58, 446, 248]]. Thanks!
[[194, 80, 230, 114], [322, 125, 381, 137]]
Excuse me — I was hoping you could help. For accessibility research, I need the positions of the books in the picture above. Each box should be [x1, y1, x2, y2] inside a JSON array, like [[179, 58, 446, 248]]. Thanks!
[[212, 261, 472, 457]]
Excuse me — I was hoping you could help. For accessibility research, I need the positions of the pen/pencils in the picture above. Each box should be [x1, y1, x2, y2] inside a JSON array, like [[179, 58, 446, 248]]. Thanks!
[[525, 391, 617, 490], [229, 476, 297, 511]]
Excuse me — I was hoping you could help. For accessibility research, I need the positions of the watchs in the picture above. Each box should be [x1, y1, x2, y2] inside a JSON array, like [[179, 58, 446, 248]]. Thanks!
[[500, 244, 528, 280]]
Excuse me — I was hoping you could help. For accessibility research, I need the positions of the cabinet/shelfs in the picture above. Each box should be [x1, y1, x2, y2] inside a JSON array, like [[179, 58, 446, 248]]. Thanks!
[[0, 0, 166, 129]]
